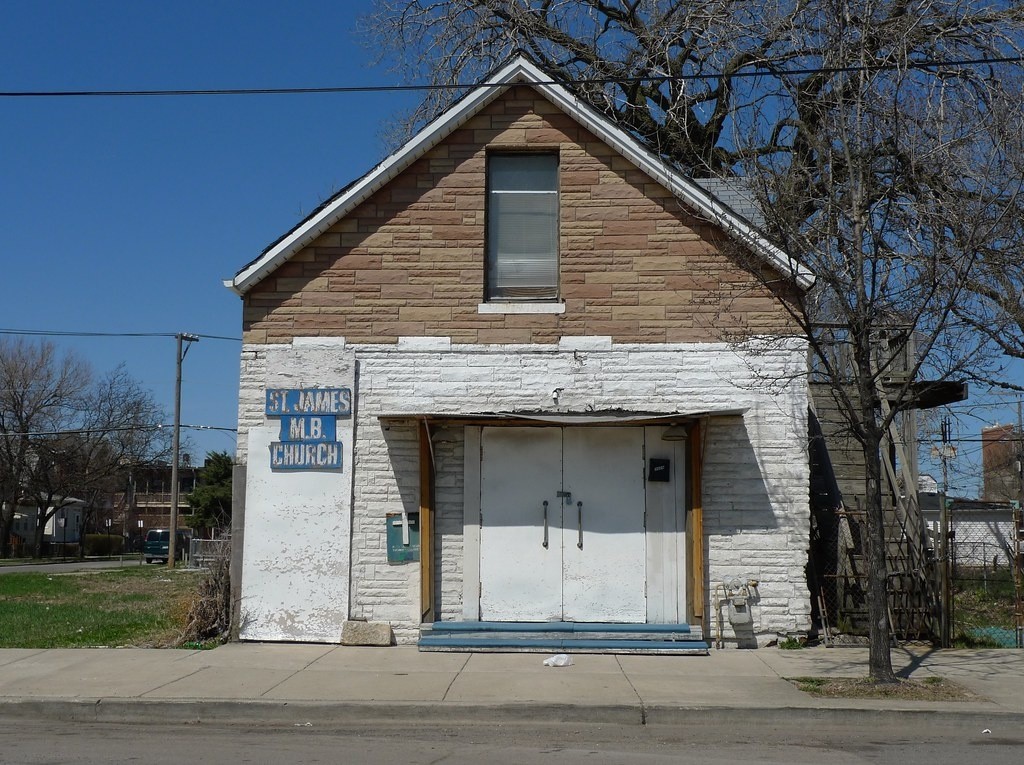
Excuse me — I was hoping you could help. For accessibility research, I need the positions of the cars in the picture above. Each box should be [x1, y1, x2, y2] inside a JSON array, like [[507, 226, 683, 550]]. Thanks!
[[144, 529, 189, 564]]
